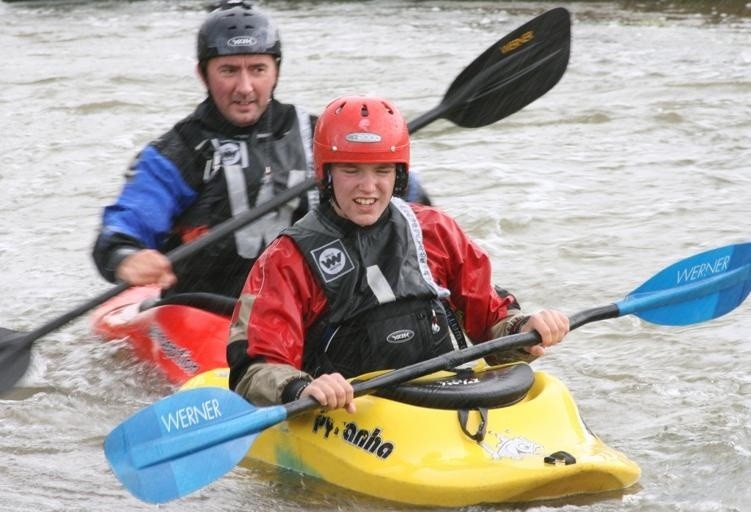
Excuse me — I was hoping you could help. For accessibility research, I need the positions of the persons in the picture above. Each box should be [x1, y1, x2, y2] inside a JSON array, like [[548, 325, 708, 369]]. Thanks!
[[90, 0, 436, 299], [225, 92, 571, 415]]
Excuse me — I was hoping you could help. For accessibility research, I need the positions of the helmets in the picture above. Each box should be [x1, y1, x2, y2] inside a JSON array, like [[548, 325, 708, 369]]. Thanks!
[[313, 94, 409, 197], [197, 4, 282, 57]]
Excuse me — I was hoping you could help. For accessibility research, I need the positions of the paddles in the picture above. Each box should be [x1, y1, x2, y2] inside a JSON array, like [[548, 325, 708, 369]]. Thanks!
[[1, 8, 571, 395], [104, 244, 750, 504]]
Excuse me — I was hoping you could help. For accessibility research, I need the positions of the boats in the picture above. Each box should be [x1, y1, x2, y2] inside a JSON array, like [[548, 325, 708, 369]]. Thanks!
[[88, 282, 642, 507]]
